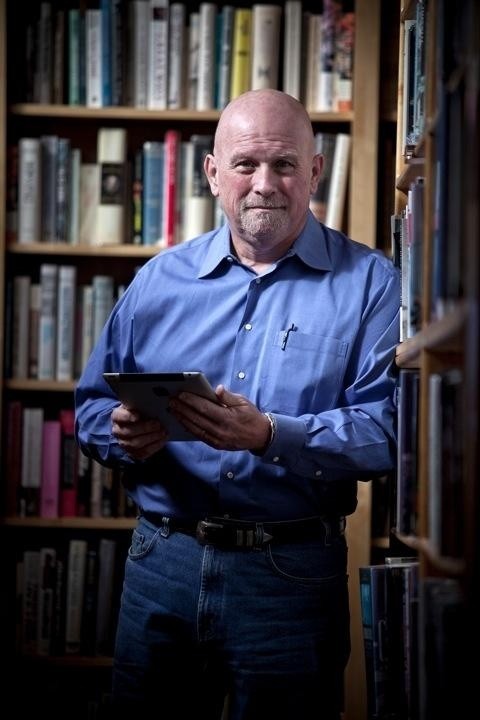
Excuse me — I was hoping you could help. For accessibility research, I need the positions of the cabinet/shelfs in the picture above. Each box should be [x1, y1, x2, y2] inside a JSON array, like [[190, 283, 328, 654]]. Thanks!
[[2, 0, 478, 719]]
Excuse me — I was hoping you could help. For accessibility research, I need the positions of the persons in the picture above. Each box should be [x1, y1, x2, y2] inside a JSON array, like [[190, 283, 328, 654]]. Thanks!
[[75, 88, 396, 717]]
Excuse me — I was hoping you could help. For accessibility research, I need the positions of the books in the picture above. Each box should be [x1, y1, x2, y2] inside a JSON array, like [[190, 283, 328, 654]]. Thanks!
[[20, 128, 350, 248], [16, 261, 141, 667], [30, 2, 353, 115], [355, 2, 478, 718]]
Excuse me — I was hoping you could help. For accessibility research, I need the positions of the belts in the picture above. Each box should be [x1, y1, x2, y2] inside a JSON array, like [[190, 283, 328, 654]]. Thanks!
[[137, 505, 343, 547]]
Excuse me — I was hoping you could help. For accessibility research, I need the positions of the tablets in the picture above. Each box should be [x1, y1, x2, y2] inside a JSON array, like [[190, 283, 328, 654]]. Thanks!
[[102, 370, 223, 443]]
